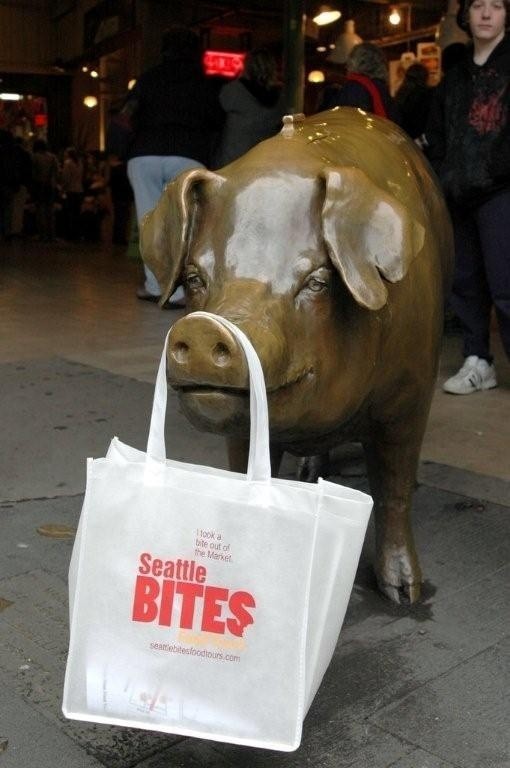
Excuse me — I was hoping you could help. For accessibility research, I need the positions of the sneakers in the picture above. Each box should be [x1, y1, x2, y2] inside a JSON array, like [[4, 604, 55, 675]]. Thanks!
[[444, 357, 499, 393], [136, 287, 186, 309]]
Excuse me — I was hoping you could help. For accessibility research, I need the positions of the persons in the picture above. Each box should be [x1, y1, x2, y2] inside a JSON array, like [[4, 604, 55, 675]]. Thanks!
[[411, 1, 509, 396], [396, 60, 434, 140], [440, 42, 467, 75], [339, 41, 393, 124], [124, 25, 223, 310], [0, 114, 128, 246], [213, 41, 296, 171]]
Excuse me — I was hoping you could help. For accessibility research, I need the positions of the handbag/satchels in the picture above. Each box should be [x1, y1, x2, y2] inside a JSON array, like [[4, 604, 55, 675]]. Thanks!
[[62, 436, 375, 752]]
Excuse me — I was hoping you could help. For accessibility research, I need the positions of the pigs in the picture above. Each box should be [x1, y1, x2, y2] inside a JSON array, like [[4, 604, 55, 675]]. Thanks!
[[138, 105, 456, 607]]
